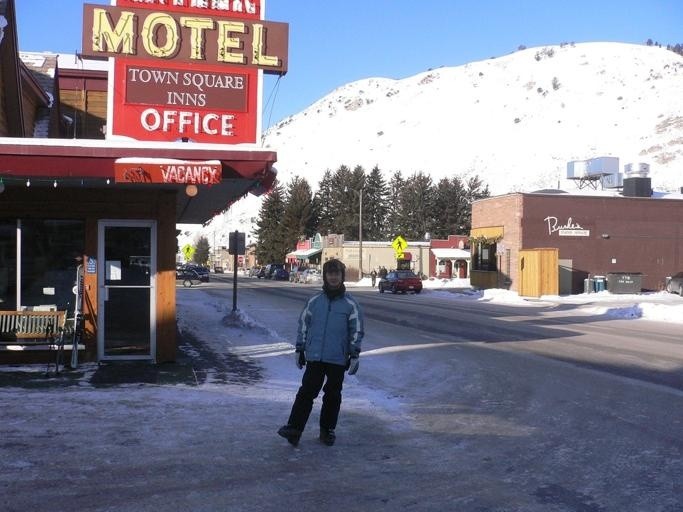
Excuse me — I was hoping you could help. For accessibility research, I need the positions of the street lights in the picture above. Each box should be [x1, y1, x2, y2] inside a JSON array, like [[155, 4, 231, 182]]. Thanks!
[[343, 185, 362, 283]]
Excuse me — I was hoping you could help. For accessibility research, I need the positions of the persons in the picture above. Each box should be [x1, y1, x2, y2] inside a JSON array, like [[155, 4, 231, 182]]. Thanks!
[[379, 266, 387, 276], [276, 257, 364, 447], [369, 268, 376, 288]]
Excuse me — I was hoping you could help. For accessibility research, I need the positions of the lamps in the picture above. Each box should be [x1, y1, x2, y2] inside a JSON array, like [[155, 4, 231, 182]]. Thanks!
[[0, 177, 110, 193], [185, 184, 199, 198]]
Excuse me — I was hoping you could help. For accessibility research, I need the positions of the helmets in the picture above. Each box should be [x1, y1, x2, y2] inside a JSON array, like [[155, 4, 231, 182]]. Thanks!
[[324, 260, 346, 280]]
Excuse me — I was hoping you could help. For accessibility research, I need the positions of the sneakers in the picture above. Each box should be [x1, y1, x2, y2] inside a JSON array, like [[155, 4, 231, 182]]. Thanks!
[[277, 425, 301, 445], [320, 427, 336, 445]]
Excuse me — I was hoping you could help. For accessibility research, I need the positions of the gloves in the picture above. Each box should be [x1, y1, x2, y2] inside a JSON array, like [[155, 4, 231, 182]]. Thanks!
[[296, 346, 306, 369], [345, 353, 359, 375]]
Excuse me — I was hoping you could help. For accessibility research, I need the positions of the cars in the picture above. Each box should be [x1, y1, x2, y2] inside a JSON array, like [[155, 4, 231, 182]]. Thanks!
[[377, 269, 422, 295], [175, 261, 209, 283], [214, 266, 223, 273], [249, 264, 322, 285]]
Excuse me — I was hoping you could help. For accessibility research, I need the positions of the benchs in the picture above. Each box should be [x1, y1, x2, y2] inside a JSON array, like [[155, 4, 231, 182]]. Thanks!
[[0, 310, 68, 378]]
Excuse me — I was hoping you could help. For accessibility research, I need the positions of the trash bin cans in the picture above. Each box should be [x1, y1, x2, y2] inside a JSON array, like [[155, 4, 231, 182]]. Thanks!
[[593, 277, 606, 293]]
